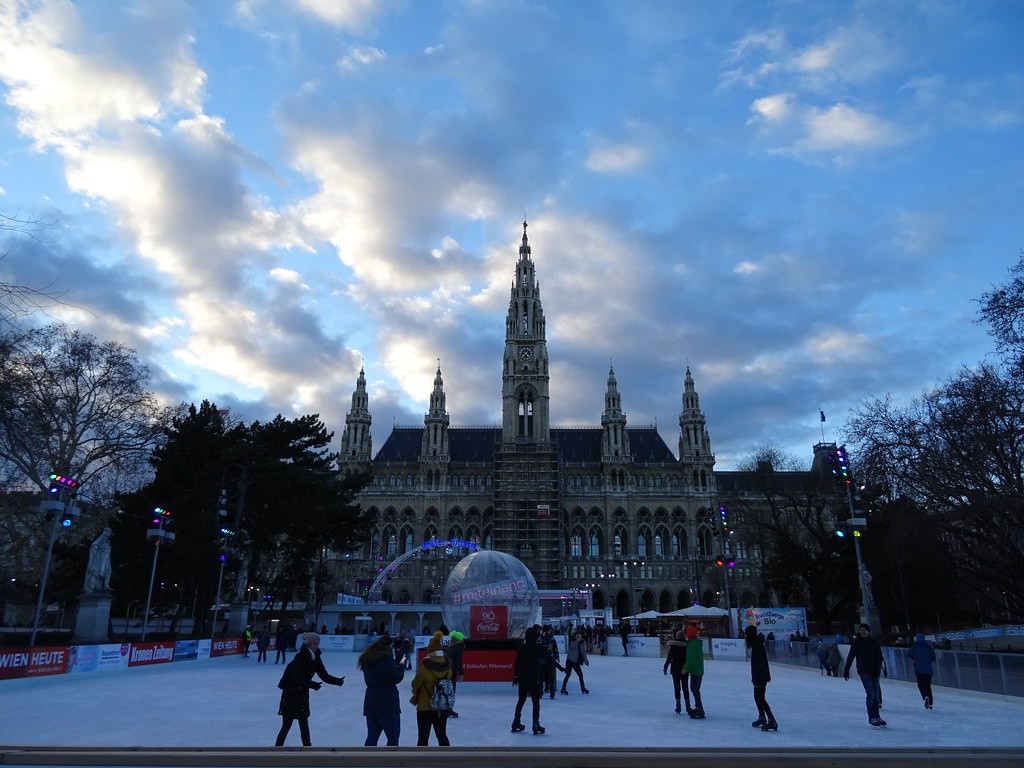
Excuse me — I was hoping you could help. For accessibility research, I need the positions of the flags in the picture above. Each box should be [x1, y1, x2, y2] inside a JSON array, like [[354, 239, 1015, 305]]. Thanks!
[[820, 410, 826, 422]]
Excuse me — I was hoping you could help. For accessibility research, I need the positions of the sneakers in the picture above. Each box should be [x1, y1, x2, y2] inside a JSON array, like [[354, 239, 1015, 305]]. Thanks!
[[533, 723, 545, 735], [511, 721, 525, 731]]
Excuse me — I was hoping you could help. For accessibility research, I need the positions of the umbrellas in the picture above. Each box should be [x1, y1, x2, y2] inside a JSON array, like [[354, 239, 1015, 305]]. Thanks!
[[622, 603, 729, 621]]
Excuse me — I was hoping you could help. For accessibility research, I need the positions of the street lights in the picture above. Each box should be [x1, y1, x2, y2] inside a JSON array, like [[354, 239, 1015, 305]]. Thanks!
[[623, 559, 646, 634], [601, 573, 615, 607], [829, 450, 878, 636], [561, 595, 572, 616], [28, 473, 82, 645], [708, 505, 737, 638], [139, 506, 177, 641], [210, 526, 236, 639], [570, 584, 600, 613]]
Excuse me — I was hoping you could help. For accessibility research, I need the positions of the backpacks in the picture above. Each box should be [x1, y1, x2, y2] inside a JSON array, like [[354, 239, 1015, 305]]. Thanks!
[[423, 669, 455, 710]]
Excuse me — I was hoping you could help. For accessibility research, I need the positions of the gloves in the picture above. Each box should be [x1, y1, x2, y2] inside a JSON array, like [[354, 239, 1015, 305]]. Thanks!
[[844, 669, 850, 682], [883, 669, 888, 678]]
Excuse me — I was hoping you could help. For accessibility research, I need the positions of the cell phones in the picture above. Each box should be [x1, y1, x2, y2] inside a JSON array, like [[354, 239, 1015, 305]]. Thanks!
[[400, 656, 407, 664]]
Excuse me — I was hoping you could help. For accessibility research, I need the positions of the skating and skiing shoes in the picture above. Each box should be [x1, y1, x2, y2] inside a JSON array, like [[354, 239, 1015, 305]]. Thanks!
[[761, 720, 778, 732], [447, 710, 458, 718], [691, 709, 706, 719], [675, 708, 681, 714], [686, 707, 693, 714], [752, 719, 767, 728]]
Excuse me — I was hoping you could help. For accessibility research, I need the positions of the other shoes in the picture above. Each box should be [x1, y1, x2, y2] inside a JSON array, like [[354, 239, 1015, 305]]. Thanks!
[[561, 689, 568, 696], [869, 718, 880, 730], [878, 718, 888, 729], [924, 697, 933, 710], [581, 688, 589, 694]]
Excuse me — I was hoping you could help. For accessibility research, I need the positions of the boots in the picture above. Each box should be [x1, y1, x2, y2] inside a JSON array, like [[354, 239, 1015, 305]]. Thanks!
[[404, 663, 411, 670]]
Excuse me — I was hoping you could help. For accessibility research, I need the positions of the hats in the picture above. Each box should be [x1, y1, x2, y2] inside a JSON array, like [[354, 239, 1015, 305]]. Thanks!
[[246, 625, 251, 628], [677, 631, 685, 639], [859, 623, 871, 633], [303, 632, 319, 645], [686, 626, 697, 638], [427, 631, 443, 651], [916, 633, 926, 640]]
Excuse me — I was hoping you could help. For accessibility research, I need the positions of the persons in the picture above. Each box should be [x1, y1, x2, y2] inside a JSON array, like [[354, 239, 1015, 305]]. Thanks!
[[242, 625, 251, 657], [789, 630, 809, 665], [561, 631, 589, 695], [275, 622, 317, 664], [664, 630, 693, 715], [511, 625, 567, 734], [410, 631, 464, 746], [737, 625, 778, 731], [256, 624, 270, 662], [941, 639, 964, 683], [359, 631, 415, 747], [908, 632, 936, 710], [321, 625, 328, 634], [619, 619, 679, 657], [815, 634, 823, 643], [335, 620, 389, 635], [816, 642, 841, 679], [423, 624, 449, 635], [550, 621, 617, 656], [680, 626, 705, 719], [894, 635, 914, 648], [276, 632, 344, 746], [844, 623, 888, 731]]
